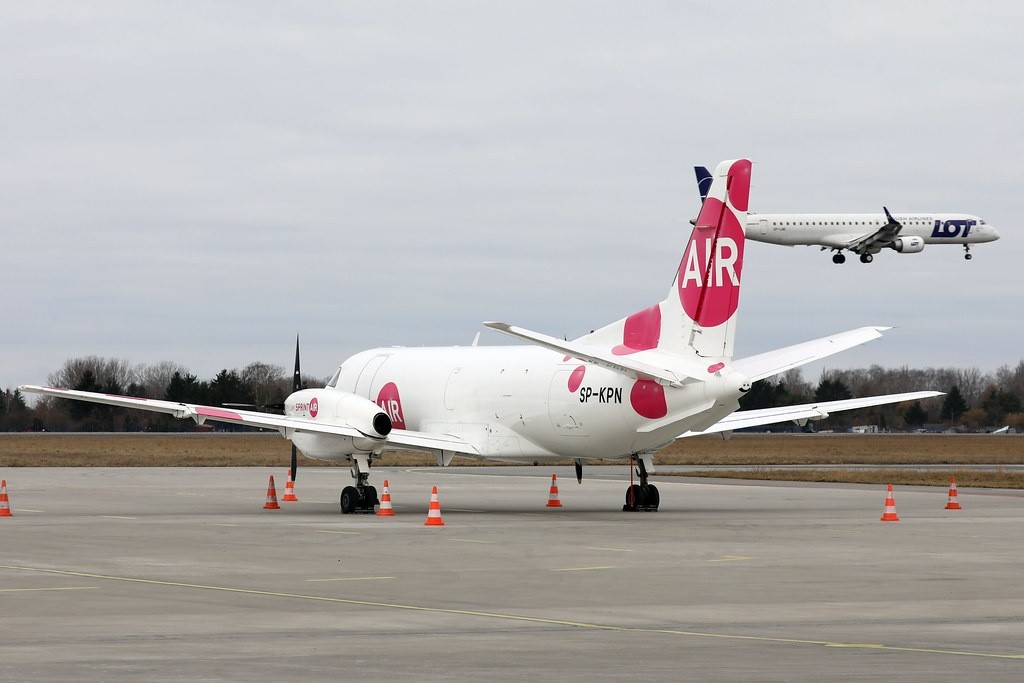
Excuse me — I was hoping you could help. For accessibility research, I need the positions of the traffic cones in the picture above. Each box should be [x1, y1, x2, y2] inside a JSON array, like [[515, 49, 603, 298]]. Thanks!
[[375, 479, 395, 516], [421, 485, 445, 526], [879, 484, 901, 522], [544, 473, 563, 507], [944, 475, 962, 510], [262, 475, 281, 509], [0, 479, 14, 517], [281, 469, 298, 502]]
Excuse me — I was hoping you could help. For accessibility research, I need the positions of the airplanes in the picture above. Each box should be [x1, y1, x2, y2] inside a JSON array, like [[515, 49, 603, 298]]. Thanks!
[[17, 157, 948, 512], [687, 165, 1001, 264]]
